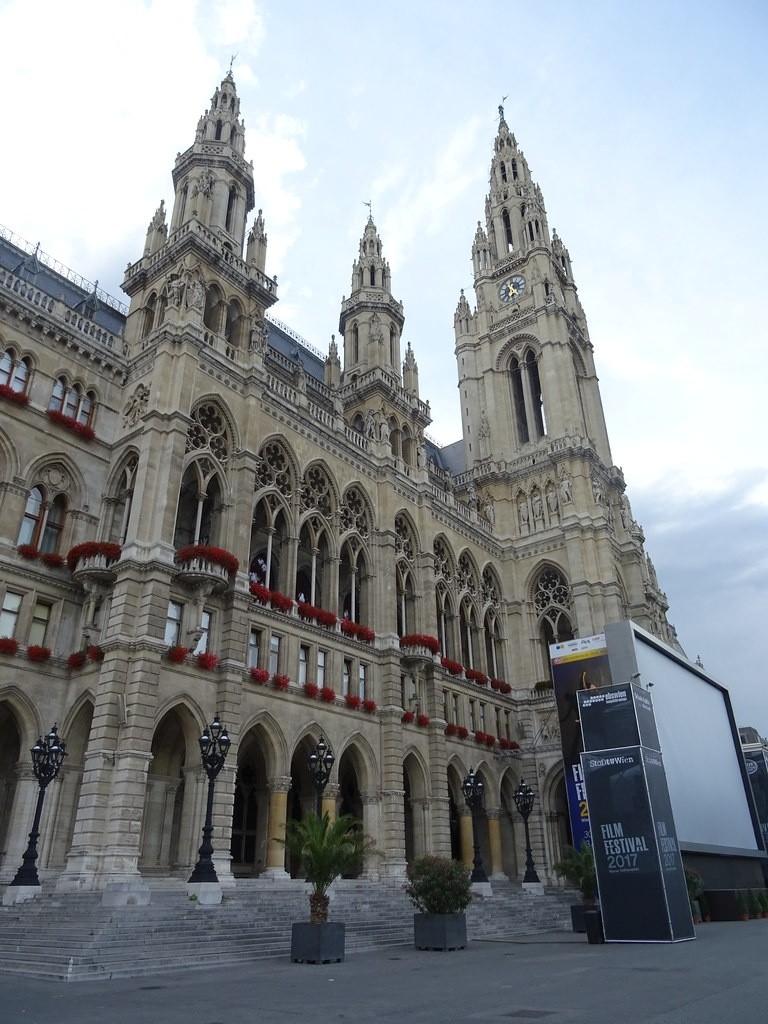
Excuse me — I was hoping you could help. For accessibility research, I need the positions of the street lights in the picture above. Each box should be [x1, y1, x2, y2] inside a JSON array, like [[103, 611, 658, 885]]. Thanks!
[[7, 720, 73, 887], [185, 709, 232, 883], [303, 731, 337, 819], [511, 775, 542, 882], [461, 765, 492, 883]]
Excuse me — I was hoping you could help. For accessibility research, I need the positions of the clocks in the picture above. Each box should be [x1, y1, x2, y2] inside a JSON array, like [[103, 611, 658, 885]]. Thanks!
[[499, 275, 525, 304]]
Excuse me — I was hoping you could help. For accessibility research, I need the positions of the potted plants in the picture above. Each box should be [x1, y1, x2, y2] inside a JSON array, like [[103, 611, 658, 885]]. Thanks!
[[733, 888, 768, 921], [552, 840, 602, 934], [684, 869, 711, 926], [402, 854, 472, 951], [271, 810, 386, 964]]
